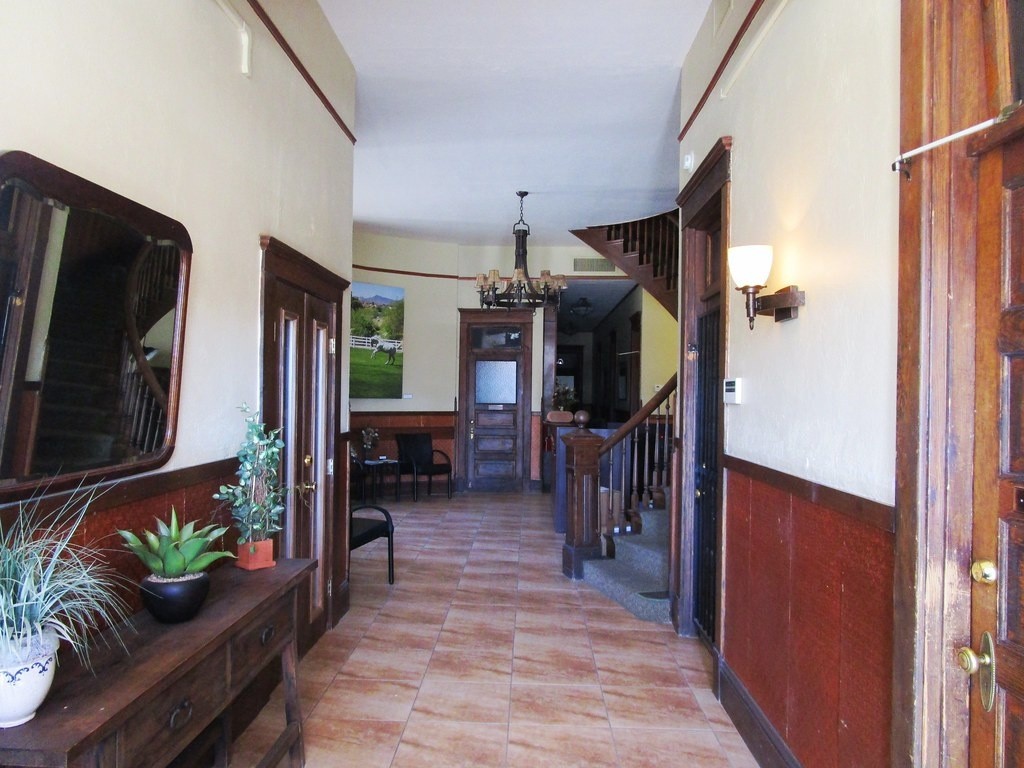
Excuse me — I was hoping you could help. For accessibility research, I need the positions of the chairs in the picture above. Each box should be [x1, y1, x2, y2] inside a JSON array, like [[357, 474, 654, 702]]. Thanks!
[[350, 458, 366, 504], [395, 433, 452, 502], [347, 503, 394, 585]]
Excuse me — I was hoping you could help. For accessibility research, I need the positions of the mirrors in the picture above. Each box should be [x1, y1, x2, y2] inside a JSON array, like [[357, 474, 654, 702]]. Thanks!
[[0, 150, 193, 505]]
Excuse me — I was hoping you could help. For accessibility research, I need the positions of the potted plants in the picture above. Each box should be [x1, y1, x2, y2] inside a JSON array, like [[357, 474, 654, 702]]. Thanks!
[[115, 503, 240, 625], [0, 471, 161, 728], [212, 401, 289, 571]]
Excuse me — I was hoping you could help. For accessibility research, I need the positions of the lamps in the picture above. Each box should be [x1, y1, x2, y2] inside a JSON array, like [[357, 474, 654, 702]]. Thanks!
[[473, 190, 568, 317], [569, 298, 594, 317], [727, 245, 805, 330]]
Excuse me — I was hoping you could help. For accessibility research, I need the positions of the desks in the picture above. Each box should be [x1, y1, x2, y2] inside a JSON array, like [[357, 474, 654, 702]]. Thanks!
[[362, 461, 401, 506], [0, 558, 318, 768]]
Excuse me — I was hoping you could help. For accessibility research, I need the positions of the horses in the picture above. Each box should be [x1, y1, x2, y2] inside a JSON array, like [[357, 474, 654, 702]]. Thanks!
[[369, 335, 396, 365]]
